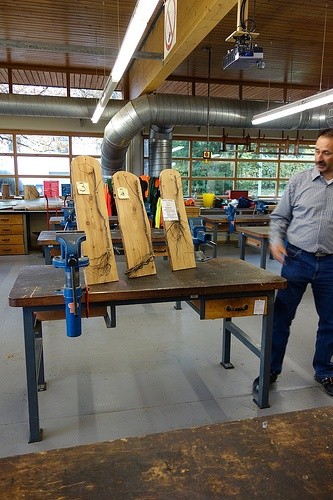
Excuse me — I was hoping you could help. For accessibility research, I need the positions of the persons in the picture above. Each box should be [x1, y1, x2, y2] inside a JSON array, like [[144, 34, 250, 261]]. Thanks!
[[254, 129, 333, 395]]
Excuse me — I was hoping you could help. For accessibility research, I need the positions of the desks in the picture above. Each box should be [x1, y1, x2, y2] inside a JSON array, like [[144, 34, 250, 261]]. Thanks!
[[49, 216, 153, 231], [37, 229, 168, 265], [200, 214, 271, 258], [201, 209, 273, 214], [236, 226, 270, 269], [8, 259, 288, 443]]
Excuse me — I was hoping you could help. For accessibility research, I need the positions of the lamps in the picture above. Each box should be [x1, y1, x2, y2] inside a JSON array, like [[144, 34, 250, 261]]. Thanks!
[[252, 4, 333, 126], [91, 0, 160, 123]]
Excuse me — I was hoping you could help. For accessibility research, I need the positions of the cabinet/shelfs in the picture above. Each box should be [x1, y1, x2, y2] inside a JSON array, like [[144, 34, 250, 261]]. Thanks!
[[0, 199, 64, 256], [184, 206, 200, 218]]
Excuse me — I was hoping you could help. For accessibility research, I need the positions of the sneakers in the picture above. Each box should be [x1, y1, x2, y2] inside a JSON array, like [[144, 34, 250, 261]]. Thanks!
[[253, 374, 277, 393], [313, 375, 333, 395]]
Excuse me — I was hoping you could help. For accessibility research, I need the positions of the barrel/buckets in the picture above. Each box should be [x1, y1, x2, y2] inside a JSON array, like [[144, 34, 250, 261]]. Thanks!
[[202, 193, 215, 208]]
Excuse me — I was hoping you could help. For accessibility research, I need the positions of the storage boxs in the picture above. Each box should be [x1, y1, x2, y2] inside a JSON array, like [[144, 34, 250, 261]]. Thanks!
[[229, 190, 248, 199]]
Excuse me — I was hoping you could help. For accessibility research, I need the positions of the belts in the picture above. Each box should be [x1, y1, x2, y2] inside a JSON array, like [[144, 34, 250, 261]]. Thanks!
[[287, 242, 333, 258]]
